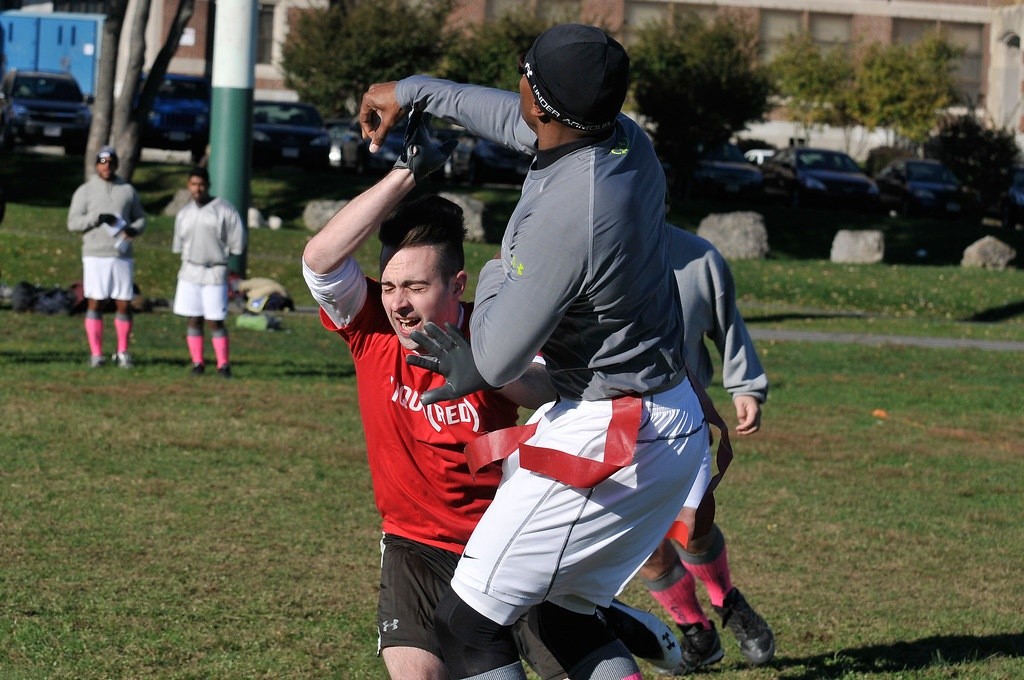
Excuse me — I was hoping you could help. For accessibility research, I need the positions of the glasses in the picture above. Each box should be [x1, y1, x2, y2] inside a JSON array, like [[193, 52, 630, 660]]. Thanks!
[[516, 48, 549, 124]]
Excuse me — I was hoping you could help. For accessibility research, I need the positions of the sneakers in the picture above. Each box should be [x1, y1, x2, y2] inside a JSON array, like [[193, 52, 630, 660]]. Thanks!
[[596, 597, 681, 672], [670, 622, 724, 674], [709, 587, 772, 666]]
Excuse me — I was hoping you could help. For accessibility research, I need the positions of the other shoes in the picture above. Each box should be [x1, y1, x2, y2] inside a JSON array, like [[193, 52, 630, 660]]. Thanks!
[[115, 352, 134, 370], [190, 364, 205, 374], [217, 366, 231, 379], [90, 356, 104, 367]]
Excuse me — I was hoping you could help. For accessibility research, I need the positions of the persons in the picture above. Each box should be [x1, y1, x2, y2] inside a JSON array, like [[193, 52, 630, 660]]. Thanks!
[[644, 220, 777, 669], [67, 145, 147, 370], [359, 23, 709, 680], [302, 102, 573, 680], [172, 167, 246, 376]]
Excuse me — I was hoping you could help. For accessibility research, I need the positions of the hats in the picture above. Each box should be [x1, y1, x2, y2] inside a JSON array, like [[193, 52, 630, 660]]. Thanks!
[[96, 145, 118, 165], [523, 21, 631, 131]]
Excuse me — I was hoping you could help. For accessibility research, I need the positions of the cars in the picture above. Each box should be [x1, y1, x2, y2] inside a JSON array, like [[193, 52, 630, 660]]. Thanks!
[[658, 136, 761, 204], [133, 71, 210, 162], [743, 150, 774, 166], [871, 158, 983, 225], [329, 108, 532, 191], [253, 100, 333, 181], [0, 65, 97, 156], [1000, 166, 1024, 207], [762, 149, 879, 217]]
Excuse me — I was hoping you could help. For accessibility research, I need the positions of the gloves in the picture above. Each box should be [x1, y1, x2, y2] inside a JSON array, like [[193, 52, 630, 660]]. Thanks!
[[404, 320, 507, 408], [100, 213, 115, 224], [390, 95, 462, 186], [126, 227, 137, 240]]
